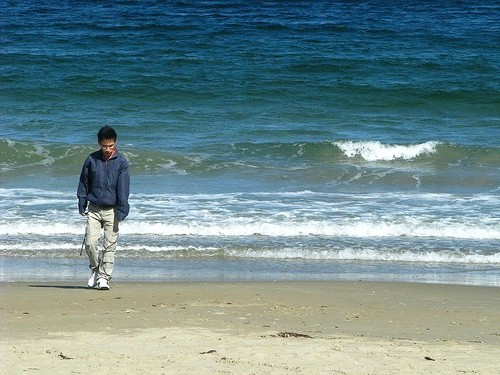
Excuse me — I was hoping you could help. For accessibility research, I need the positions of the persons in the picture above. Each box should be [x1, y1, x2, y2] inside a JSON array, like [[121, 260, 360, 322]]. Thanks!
[[77, 125, 130, 290]]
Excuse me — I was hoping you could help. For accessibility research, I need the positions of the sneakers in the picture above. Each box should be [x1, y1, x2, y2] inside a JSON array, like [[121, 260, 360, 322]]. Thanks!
[[97, 277, 110, 290], [87, 270, 98, 287]]
